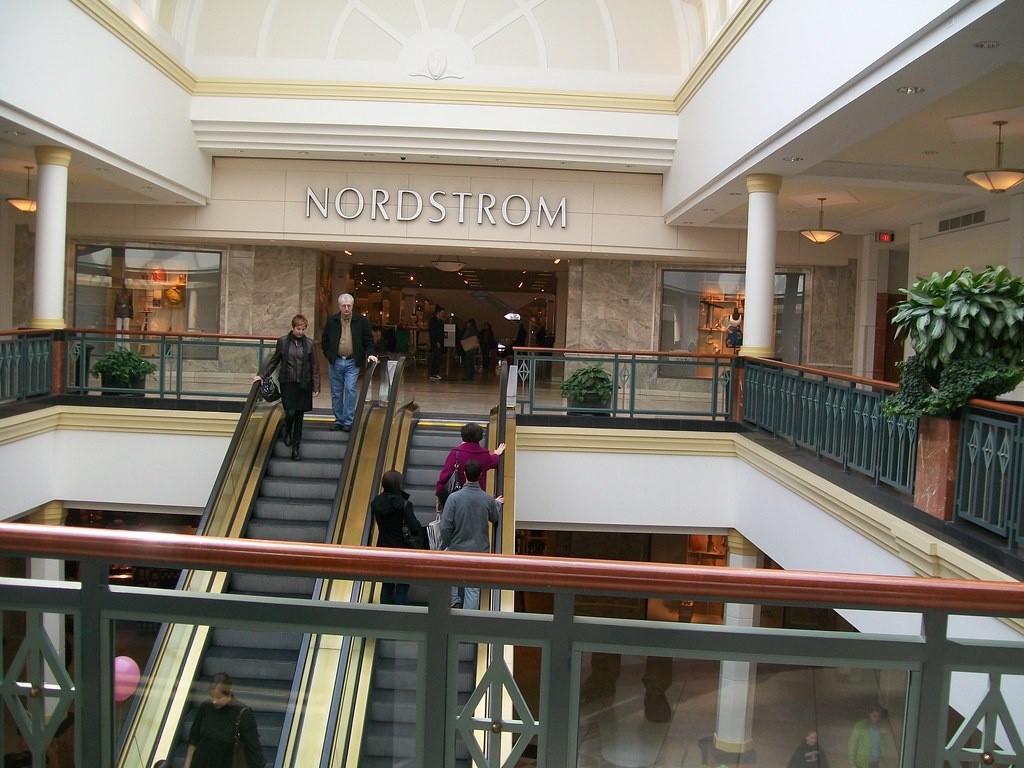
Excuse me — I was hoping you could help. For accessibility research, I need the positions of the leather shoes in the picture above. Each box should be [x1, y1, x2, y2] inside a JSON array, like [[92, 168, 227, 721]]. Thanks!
[[330, 424, 351, 432]]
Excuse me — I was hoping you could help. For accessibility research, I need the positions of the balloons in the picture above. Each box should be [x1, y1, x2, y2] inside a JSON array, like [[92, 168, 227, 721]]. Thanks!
[[115, 656, 141, 702]]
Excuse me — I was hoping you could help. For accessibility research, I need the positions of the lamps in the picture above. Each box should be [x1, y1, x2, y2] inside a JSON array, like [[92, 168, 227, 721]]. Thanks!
[[430, 255, 467, 273], [6, 165, 37, 213], [961, 119, 1024, 197], [799, 197, 843, 247]]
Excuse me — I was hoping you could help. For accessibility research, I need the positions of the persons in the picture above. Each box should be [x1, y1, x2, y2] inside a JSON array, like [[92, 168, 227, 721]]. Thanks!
[[183, 672, 268, 768], [435, 422, 507, 514], [114, 284, 130, 352], [728, 307, 743, 347], [536, 327, 546, 355], [786, 730, 831, 768], [321, 294, 378, 433], [458, 318, 493, 383], [848, 705, 891, 768], [252, 314, 321, 461], [517, 324, 527, 355], [427, 304, 445, 380], [370, 470, 428, 605], [439, 459, 505, 610]]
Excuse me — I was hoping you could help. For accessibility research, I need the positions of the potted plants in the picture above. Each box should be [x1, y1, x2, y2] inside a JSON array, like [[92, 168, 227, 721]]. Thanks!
[[559, 363, 622, 417], [88, 344, 158, 398]]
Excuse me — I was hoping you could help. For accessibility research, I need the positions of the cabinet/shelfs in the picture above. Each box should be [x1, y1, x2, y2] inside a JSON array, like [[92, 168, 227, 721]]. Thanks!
[[102, 256, 192, 361], [695, 280, 777, 378]]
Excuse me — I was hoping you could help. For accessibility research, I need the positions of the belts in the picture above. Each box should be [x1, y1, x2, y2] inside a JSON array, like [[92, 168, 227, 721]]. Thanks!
[[337, 355, 354, 360]]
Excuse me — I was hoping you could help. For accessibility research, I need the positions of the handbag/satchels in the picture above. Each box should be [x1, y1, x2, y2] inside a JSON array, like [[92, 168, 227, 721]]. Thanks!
[[401, 500, 426, 549], [460, 336, 481, 352], [231, 708, 267, 768], [259, 377, 281, 402], [427, 513, 442, 550], [438, 451, 461, 506]]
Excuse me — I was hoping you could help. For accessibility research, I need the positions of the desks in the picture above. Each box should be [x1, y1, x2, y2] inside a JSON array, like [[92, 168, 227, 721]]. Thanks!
[[403, 325, 430, 353]]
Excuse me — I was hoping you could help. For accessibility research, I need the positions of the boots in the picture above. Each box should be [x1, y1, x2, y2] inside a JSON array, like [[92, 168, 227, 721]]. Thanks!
[[284, 416, 292, 445], [291, 432, 302, 461]]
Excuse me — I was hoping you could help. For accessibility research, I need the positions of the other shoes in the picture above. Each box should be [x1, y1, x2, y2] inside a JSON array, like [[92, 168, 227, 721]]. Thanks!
[[462, 375, 474, 381], [643, 691, 671, 724], [579, 676, 615, 703]]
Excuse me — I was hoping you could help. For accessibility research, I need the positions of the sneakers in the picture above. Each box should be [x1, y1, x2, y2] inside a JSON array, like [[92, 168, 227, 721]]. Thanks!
[[429, 375, 442, 379]]
